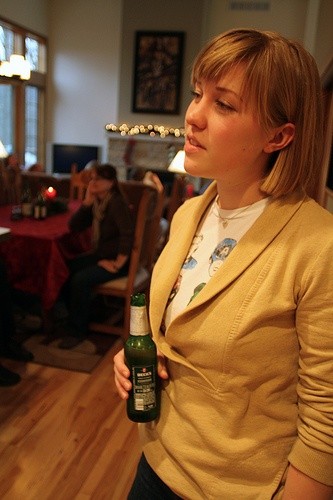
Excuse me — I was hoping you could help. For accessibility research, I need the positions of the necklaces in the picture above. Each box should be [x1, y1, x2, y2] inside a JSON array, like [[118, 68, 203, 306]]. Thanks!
[[214, 200, 255, 229]]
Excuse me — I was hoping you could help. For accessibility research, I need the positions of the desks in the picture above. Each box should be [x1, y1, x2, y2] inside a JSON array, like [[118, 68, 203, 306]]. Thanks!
[[0, 198, 93, 309]]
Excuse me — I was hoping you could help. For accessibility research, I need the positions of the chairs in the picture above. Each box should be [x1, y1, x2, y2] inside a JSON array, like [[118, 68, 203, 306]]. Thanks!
[[0, 167, 186, 335]]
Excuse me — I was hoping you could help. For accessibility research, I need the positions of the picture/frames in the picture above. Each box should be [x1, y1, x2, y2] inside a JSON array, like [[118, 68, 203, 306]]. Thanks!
[[130, 30, 186, 115]]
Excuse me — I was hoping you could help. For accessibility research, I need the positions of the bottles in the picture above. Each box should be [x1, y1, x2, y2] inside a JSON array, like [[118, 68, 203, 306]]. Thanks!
[[124, 292, 161, 423], [20, 177, 50, 219]]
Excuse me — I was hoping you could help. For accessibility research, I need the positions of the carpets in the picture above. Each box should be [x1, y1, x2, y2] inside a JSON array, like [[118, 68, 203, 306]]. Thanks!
[[0, 316, 126, 373]]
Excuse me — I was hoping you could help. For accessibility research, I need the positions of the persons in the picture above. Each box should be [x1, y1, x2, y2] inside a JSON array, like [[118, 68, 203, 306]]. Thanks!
[[114, 29, 333, 500], [42, 164, 133, 349]]
